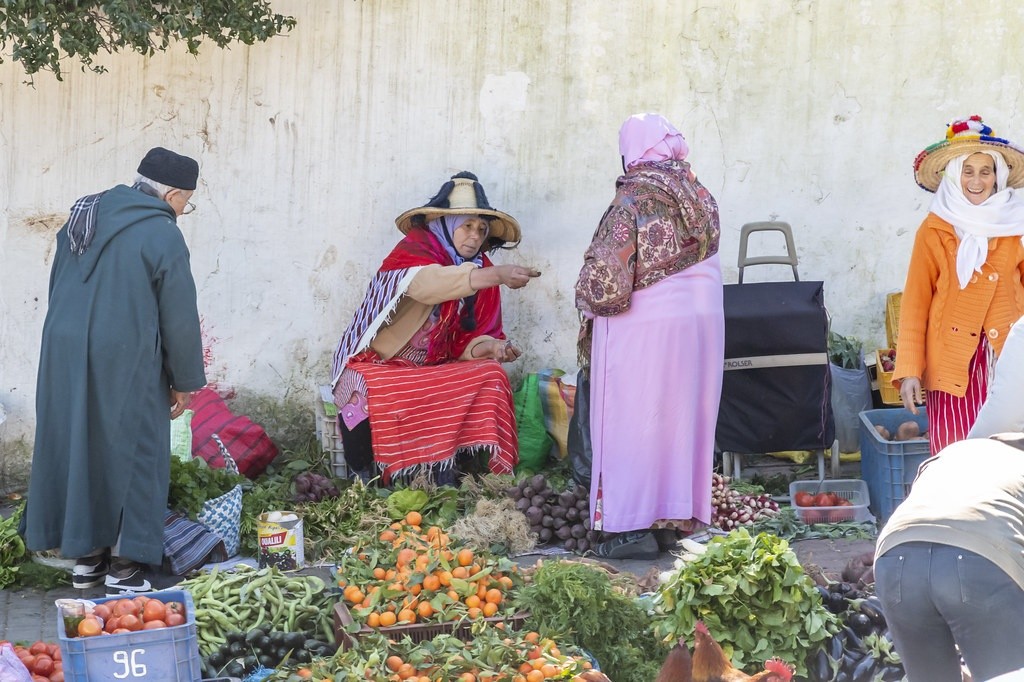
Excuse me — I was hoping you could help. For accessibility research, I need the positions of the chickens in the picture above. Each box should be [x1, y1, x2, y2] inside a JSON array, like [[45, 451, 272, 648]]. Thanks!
[[655, 619, 792, 682]]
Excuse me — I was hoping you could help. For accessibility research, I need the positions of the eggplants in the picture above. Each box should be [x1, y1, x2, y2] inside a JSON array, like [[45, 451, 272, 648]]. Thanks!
[[203, 621, 337, 677], [809, 574, 907, 682]]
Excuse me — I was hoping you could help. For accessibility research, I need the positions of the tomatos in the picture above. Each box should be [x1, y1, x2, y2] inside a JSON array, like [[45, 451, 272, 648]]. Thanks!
[[795, 491, 854, 524], [0, 596, 184, 682]]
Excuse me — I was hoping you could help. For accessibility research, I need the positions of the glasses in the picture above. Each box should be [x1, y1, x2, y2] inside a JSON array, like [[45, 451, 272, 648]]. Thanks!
[[181, 197, 196, 214]]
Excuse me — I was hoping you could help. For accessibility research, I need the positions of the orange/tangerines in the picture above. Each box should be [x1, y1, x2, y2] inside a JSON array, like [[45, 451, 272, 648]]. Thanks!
[[340, 509, 591, 682]]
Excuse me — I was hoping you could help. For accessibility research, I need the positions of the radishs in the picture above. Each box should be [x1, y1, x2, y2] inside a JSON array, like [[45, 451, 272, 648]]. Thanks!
[[655, 532, 843, 678]]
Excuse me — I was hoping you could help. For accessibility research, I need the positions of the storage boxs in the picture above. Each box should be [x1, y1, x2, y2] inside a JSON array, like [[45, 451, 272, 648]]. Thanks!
[[788, 479, 871, 527], [55, 589, 203, 682], [875, 347, 927, 406], [857, 404, 932, 530], [332, 597, 534, 660]]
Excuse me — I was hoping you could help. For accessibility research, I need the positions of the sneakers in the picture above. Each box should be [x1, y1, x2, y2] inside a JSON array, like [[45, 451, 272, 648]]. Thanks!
[[72, 561, 110, 589], [656, 529, 679, 551], [593, 531, 659, 561], [105, 568, 152, 597]]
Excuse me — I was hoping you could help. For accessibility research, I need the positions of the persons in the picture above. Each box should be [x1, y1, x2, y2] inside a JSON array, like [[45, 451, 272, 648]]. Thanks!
[[574, 113, 728, 561], [890, 116, 1024, 456], [334, 171, 539, 485], [966, 312, 1024, 440], [20, 148, 207, 593], [869, 439, 1024, 682]]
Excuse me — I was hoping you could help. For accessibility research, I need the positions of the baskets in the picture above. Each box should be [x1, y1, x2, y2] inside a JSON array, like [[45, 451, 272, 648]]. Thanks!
[[886, 291, 903, 348], [875, 348, 928, 405], [788, 479, 871, 523]]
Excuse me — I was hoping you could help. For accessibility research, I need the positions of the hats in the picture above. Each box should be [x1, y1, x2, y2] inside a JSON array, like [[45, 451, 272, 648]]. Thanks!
[[395, 171, 521, 243], [136, 147, 199, 191], [913, 112, 1024, 193]]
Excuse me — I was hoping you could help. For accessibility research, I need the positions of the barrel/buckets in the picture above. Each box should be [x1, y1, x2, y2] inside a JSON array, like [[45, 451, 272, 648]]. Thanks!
[[257, 511, 305, 574]]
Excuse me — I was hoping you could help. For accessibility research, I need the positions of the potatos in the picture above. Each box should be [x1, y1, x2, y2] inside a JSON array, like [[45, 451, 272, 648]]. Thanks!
[[875, 426, 890, 440], [498, 473, 610, 553], [895, 420, 918, 442]]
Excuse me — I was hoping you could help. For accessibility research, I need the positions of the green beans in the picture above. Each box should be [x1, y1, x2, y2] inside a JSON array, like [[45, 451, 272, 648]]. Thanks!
[[178, 561, 340, 672]]
